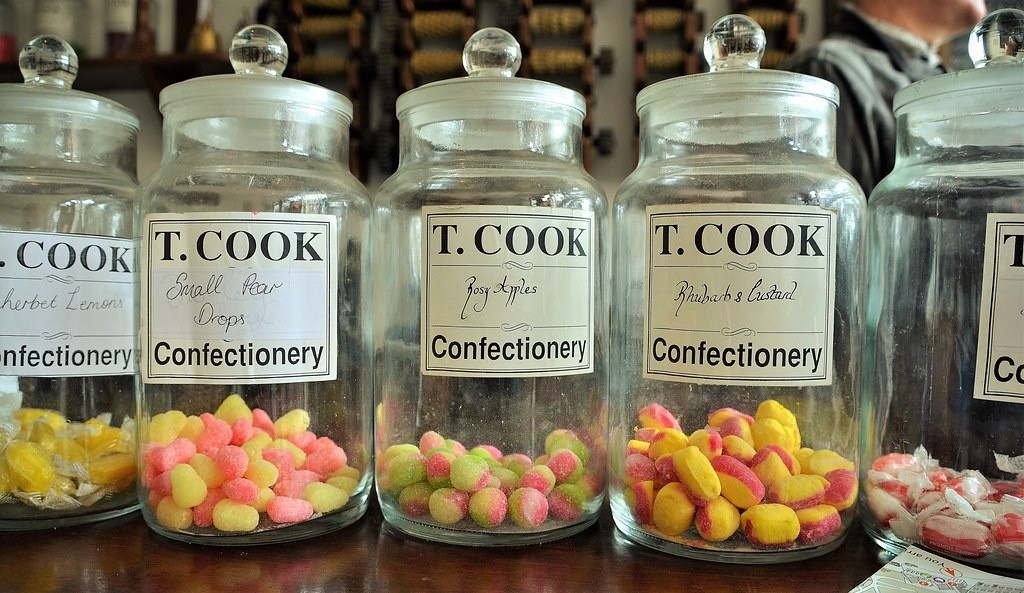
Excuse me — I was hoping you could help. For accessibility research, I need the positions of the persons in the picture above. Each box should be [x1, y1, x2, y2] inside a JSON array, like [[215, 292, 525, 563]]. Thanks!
[[779, 0, 989, 200]]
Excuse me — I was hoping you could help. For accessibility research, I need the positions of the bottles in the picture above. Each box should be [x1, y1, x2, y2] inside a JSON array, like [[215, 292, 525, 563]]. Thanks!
[[370, 30, 606, 549], [134, 23, 373, 546], [607, 15, 871, 562], [865, 6, 1024, 576], [103, 0, 134, 60], [0, 36, 140, 529], [33, 0, 75, 39]]
